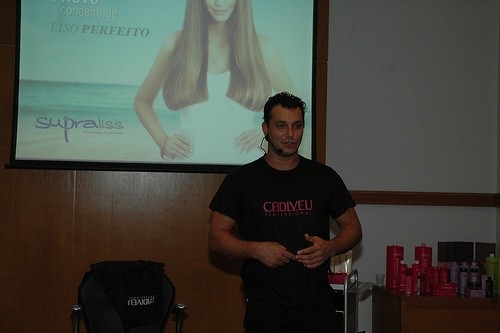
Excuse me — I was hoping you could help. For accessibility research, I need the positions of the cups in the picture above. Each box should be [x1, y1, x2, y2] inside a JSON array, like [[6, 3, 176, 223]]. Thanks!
[[375, 273, 385, 286]]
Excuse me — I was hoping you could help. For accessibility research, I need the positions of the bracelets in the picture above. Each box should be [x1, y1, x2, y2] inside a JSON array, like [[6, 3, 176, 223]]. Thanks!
[[161, 145, 176, 161]]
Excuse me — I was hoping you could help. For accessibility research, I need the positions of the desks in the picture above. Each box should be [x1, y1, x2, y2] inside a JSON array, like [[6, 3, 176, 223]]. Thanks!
[[372, 284, 500, 333]]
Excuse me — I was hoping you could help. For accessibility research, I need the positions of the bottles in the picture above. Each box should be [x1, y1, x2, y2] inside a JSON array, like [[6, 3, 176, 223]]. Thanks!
[[386, 242, 432, 296], [470, 254, 500, 297], [432, 260, 469, 295]]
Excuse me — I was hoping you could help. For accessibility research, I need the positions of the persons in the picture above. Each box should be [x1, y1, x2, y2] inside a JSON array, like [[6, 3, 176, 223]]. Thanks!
[[134, 0, 298, 164], [208, 91, 364, 333]]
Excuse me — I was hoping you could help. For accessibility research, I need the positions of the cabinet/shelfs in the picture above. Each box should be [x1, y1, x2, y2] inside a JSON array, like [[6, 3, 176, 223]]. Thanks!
[[332, 269, 358, 333]]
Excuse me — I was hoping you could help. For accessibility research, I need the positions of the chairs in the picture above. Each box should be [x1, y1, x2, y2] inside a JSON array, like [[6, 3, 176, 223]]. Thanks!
[[71, 260, 186, 333]]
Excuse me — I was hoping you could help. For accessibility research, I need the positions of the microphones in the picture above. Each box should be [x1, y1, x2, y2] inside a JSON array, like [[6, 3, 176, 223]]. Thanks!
[[266, 136, 284, 154]]
